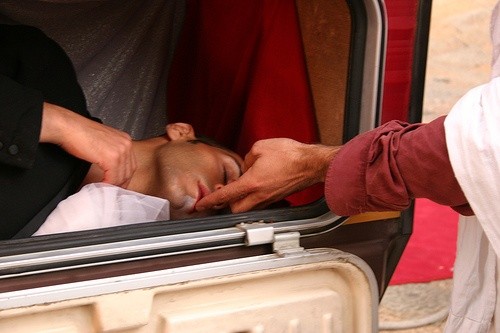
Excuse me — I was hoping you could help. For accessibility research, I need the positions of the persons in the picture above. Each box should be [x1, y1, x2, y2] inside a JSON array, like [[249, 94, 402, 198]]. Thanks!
[[195, 0, 499, 332], [0, 24, 245, 242]]
